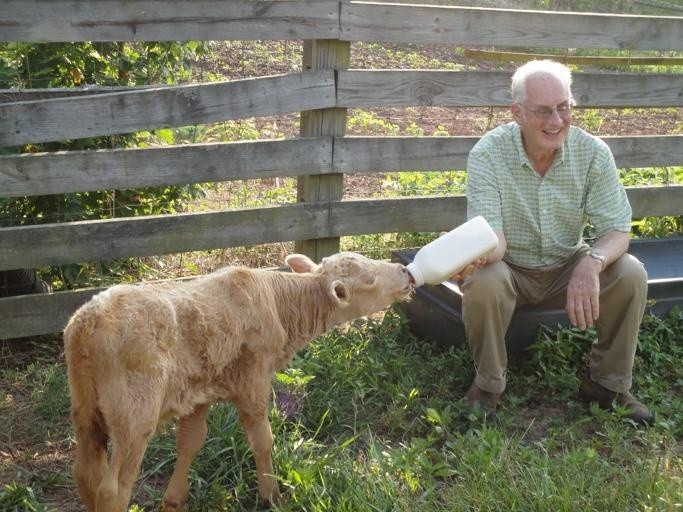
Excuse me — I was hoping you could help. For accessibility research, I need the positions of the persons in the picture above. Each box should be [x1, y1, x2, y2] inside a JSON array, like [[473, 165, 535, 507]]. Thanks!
[[438, 60, 654, 430]]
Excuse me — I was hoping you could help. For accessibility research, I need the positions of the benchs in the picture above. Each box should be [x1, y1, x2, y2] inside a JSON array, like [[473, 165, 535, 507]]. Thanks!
[[390, 236, 683, 365]]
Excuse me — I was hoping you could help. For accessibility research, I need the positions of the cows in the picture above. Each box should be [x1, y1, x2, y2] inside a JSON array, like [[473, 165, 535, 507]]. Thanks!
[[61, 249, 414, 512]]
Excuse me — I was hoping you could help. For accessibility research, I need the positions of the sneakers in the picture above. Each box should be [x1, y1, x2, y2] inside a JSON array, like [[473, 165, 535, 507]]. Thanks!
[[581, 371, 654, 430], [461, 380, 502, 419]]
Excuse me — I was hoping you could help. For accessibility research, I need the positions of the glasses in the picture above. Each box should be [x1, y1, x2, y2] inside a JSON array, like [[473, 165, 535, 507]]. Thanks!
[[521, 104, 572, 119]]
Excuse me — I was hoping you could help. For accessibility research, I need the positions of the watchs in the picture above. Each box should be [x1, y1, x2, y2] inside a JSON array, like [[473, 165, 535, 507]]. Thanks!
[[580, 248, 609, 275]]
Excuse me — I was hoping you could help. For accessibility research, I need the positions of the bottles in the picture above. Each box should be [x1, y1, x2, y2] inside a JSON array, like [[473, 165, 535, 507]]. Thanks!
[[404, 214, 501, 290]]
